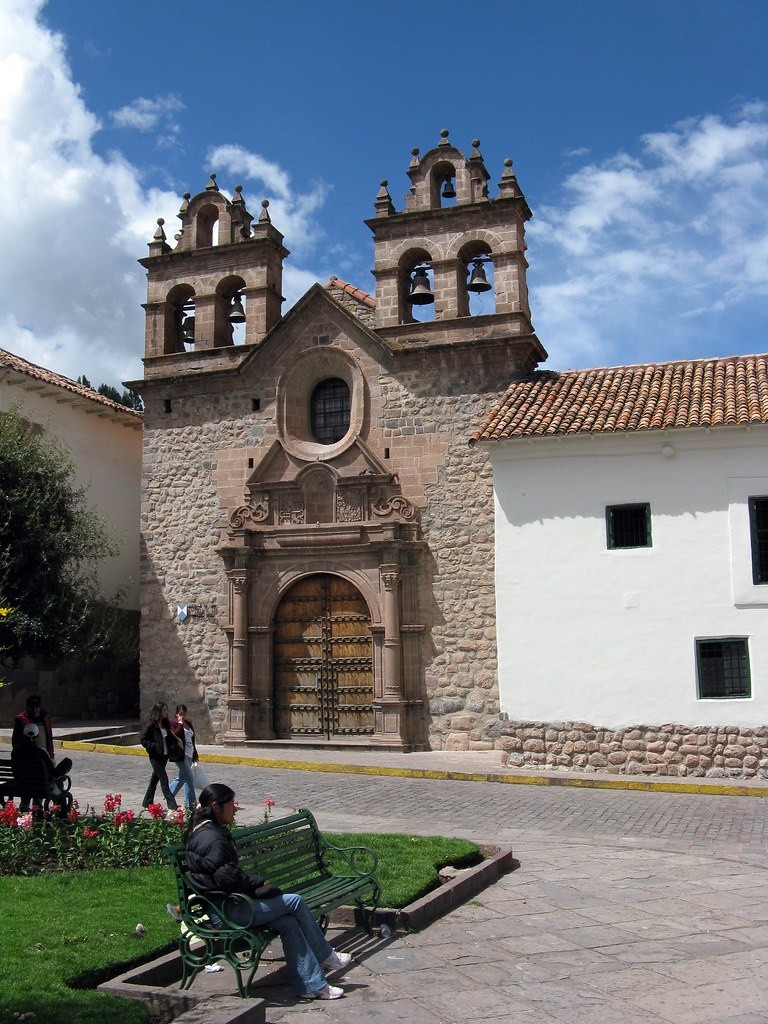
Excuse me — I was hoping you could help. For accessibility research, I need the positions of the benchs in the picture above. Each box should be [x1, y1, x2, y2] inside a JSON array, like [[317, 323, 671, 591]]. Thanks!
[[0, 759, 72, 816], [161, 806, 383, 999]]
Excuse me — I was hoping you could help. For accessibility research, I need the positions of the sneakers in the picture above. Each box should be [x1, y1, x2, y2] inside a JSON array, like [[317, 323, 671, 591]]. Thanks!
[[300, 983, 344, 999], [321, 948, 352, 970]]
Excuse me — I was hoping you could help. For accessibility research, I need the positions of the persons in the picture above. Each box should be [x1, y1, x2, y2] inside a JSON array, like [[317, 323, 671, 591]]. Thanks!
[[10, 693, 73, 818], [182, 784, 352, 1001], [141, 701, 200, 811]]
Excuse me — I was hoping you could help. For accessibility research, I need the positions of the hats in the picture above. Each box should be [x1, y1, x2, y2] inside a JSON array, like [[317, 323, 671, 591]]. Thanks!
[[23, 723, 40, 737], [27, 694, 42, 706]]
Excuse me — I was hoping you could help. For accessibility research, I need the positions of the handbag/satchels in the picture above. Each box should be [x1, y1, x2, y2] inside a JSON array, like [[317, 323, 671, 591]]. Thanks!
[[192, 759, 209, 791]]
